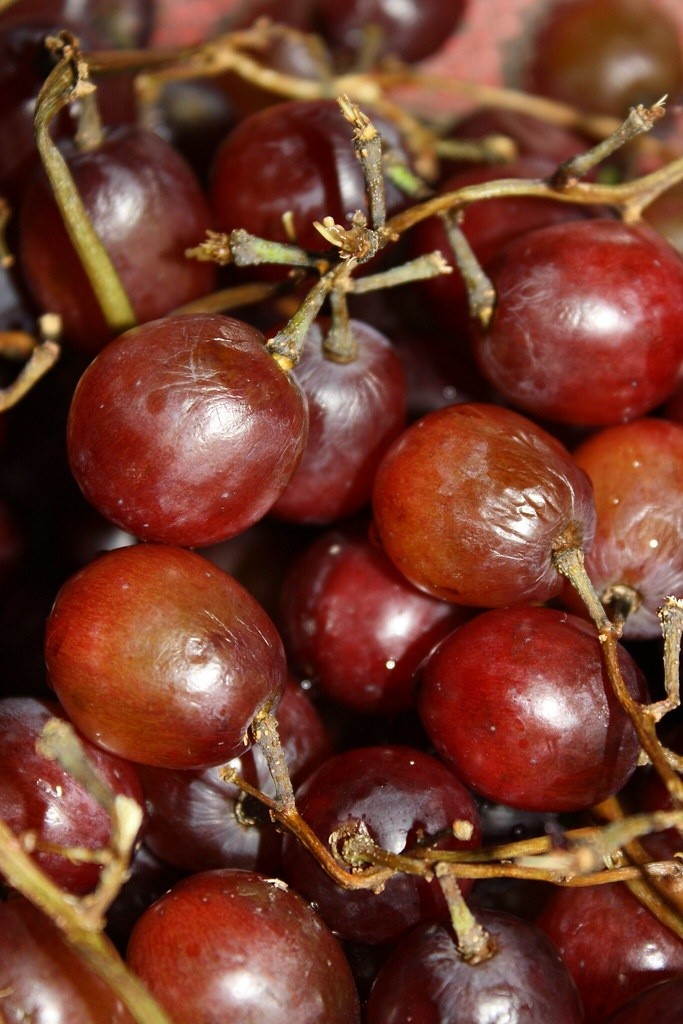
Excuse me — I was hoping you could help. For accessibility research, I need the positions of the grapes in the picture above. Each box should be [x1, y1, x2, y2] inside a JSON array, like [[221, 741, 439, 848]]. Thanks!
[[0, 0, 683, 1024]]
